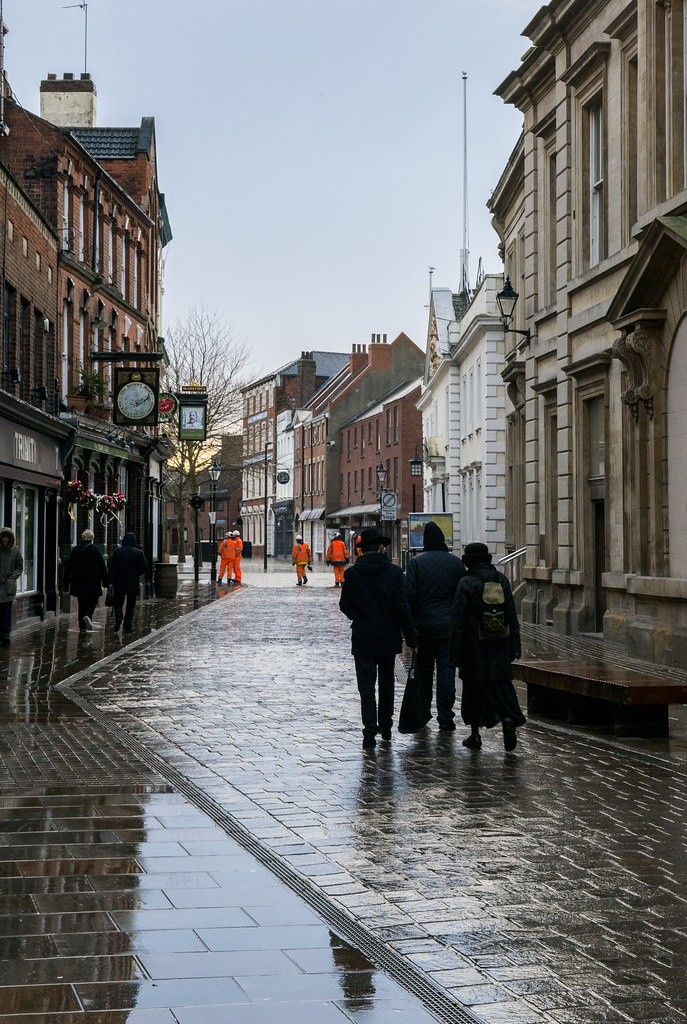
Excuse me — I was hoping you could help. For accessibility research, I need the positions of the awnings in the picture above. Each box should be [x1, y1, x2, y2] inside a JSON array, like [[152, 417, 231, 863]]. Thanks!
[[300, 504, 382, 521]]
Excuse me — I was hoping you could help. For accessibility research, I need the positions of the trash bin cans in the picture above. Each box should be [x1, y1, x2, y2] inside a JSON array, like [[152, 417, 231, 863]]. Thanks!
[[243, 541, 253, 558], [153, 563, 179, 599], [206, 542, 218, 562]]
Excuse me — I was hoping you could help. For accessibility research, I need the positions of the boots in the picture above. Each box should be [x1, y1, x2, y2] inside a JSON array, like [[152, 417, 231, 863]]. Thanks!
[[341, 582, 343, 587], [303, 576, 308, 584], [296, 581, 302, 585], [333, 582, 339, 587]]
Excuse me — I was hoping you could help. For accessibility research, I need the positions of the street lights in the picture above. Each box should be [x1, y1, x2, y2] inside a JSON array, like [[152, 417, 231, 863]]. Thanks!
[[376, 461, 388, 552], [208, 460, 222, 581]]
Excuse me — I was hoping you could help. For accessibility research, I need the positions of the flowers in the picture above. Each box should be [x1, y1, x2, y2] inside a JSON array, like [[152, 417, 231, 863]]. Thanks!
[[61, 481, 129, 514]]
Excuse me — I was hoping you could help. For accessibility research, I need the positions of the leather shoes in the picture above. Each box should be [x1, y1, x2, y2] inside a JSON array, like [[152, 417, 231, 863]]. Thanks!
[[502, 722, 517, 752], [463, 733, 482, 747]]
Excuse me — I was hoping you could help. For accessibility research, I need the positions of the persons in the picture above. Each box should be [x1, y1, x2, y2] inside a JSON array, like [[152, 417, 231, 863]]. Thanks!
[[450, 542, 526, 750], [107, 532, 149, 633], [408, 521, 467, 730], [64, 530, 107, 633], [325, 531, 362, 588], [339, 529, 421, 747], [216, 530, 243, 583], [0, 527, 23, 646], [292, 535, 311, 586]]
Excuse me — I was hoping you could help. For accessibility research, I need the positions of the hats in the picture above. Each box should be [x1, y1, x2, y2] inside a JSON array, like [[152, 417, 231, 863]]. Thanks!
[[462, 543, 492, 566], [423, 521, 446, 542], [355, 529, 392, 548]]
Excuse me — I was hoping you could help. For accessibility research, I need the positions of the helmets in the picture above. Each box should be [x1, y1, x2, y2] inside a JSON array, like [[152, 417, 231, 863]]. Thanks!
[[225, 531, 233, 537], [295, 535, 302, 541], [349, 531, 355, 538], [334, 532, 341, 538], [233, 530, 240, 537]]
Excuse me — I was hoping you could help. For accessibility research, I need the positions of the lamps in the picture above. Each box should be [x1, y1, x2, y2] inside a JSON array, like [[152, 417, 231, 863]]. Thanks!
[[408, 444, 428, 477], [495, 274, 531, 344]]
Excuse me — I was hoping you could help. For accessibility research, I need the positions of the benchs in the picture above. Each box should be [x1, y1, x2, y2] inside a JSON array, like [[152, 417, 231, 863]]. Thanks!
[[510, 658, 687, 743]]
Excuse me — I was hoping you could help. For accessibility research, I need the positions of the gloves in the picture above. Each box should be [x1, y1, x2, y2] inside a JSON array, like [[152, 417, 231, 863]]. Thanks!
[[327, 563, 329, 566], [346, 559, 349, 564]]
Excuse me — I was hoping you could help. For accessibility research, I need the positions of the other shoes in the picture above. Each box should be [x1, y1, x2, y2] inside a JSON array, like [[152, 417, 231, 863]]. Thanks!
[[0, 636, 10, 646], [362, 726, 380, 737], [83, 616, 92, 630], [378, 726, 391, 740], [114, 618, 122, 632], [231, 579, 240, 584], [439, 722, 456, 730], [227, 578, 231, 584], [217, 579, 222, 583]]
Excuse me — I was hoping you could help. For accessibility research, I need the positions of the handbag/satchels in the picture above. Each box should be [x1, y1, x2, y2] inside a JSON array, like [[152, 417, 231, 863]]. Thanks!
[[105, 588, 112, 608], [398, 648, 423, 734]]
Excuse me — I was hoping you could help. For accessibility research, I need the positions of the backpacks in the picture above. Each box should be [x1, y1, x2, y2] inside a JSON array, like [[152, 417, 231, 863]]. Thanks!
[[467, 573, 511, 652]]
